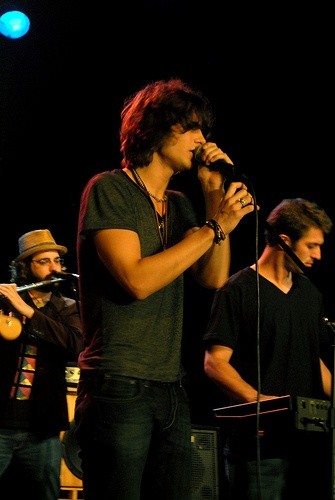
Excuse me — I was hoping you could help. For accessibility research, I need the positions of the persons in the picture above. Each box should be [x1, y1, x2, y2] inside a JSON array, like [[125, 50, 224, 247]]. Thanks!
[[200, 197, 335, 500], [0, 229, 83, 500], [75, 78, 261, 500]]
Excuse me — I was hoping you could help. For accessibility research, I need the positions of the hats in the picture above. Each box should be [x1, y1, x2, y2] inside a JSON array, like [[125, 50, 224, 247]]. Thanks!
[[15, 229, 68, 262]]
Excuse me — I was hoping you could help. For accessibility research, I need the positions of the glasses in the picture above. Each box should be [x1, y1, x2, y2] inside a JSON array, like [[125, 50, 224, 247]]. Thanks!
[[30, 258, 65, 265]]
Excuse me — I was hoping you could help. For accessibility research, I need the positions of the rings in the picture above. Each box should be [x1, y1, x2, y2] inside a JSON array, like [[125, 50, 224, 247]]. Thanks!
[[239, 198, 245, 207]]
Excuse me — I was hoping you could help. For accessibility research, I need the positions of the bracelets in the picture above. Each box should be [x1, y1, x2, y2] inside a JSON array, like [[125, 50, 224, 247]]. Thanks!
[[205, 219, 226, 244]]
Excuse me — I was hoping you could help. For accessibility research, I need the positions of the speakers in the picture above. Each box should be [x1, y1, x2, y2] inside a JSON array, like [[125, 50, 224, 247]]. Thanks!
[[59, 387, 83, 490], [187, 429, 220, 500]]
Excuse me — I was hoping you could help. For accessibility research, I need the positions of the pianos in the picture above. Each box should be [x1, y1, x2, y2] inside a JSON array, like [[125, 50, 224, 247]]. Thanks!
[[213, 394, 335, 500]]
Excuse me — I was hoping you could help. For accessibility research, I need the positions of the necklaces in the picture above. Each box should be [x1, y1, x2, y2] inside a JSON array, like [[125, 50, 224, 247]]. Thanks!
[[29, 290, 40, 303], [132, 168, 168, 251]]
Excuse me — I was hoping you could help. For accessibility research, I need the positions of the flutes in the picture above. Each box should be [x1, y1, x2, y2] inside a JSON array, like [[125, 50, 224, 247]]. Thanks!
[[0, 273, 80, 298]]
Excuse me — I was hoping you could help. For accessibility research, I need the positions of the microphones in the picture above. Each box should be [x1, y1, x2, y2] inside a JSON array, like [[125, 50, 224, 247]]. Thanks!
[[193, 145, 248, 180]]
